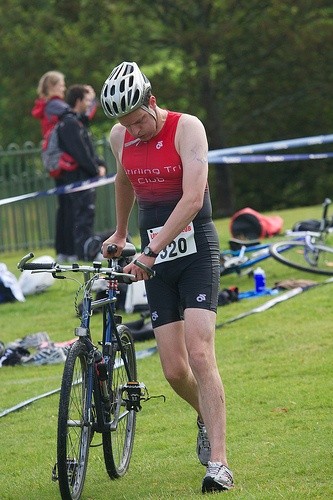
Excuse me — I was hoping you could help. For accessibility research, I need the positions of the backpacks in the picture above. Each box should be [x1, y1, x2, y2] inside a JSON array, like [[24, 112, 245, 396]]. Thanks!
[[42, 115, 83, 177]]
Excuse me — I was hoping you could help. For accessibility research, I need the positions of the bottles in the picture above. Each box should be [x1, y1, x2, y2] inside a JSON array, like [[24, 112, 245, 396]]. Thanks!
[[94, 350, 113, 401], [254, 267, 265, 293]]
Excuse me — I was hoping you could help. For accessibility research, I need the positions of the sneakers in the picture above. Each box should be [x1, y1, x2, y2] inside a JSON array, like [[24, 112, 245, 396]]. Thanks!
[[201, 461, 234, 494], [196, 415, 211, 465], [0, 331, 71, 366]]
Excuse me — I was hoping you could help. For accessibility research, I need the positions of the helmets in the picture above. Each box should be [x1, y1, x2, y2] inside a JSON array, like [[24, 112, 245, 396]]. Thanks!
[[100, 61, 151, 119]]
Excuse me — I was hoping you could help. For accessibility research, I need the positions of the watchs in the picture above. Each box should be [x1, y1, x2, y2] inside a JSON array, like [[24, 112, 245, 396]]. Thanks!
[[144, 247, 159, 258]]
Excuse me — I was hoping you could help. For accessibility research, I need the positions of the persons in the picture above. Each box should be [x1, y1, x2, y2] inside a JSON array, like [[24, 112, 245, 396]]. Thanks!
[[99, 61, 234, 493], [31, 70, 108, 264]]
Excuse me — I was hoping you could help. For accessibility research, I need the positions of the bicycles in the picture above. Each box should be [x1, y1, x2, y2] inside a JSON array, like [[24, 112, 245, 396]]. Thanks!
[[219, 198, 333, 277], [16, 242, 149, 500]]
